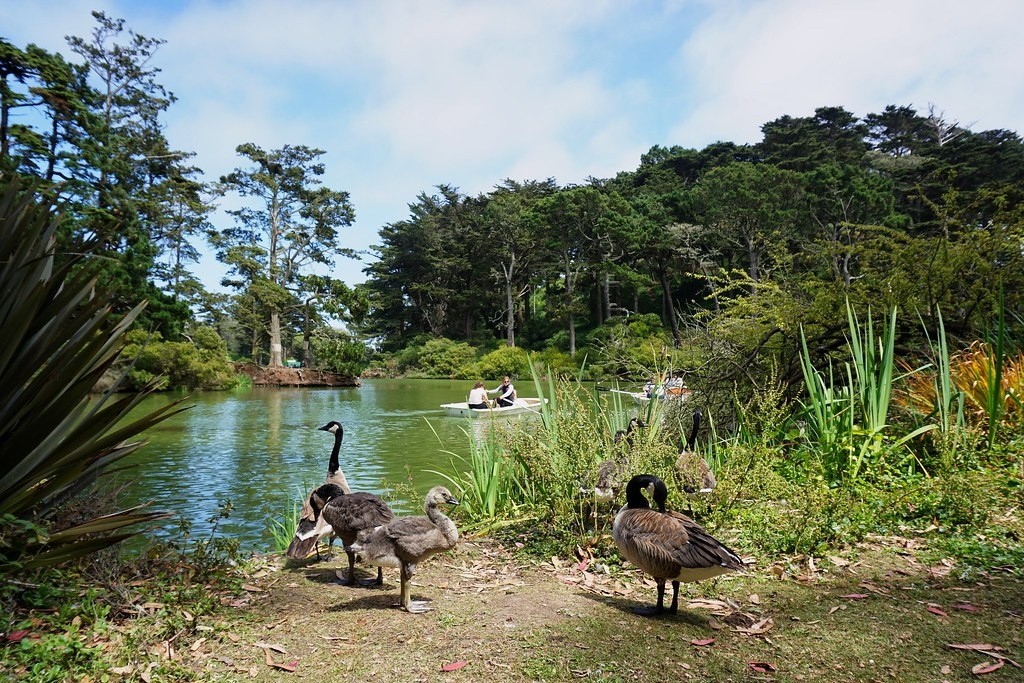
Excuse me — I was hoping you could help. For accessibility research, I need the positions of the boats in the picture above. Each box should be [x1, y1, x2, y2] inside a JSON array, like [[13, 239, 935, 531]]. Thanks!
[[609, 388, 701, 407], [438, 396, 550, 418]]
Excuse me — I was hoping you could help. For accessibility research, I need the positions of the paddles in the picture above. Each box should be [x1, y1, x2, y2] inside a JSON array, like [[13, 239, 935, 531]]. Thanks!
[[503, 397, 544, 415], [593, 386, 647, 395]]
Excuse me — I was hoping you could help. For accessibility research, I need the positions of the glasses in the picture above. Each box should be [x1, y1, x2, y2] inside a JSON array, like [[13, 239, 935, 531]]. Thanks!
[[504, 381, 509, 383]]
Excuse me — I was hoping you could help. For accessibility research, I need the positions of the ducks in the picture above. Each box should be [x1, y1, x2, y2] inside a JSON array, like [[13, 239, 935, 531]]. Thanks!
[[578, 407, 743, 620], [282, 421, 461, 615]]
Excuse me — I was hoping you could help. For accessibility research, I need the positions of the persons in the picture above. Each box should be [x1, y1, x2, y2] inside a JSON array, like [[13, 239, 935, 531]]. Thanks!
[[485, 376, 516, 408], [643, 375, 683, 400], [468, 381, 492, 410]]
